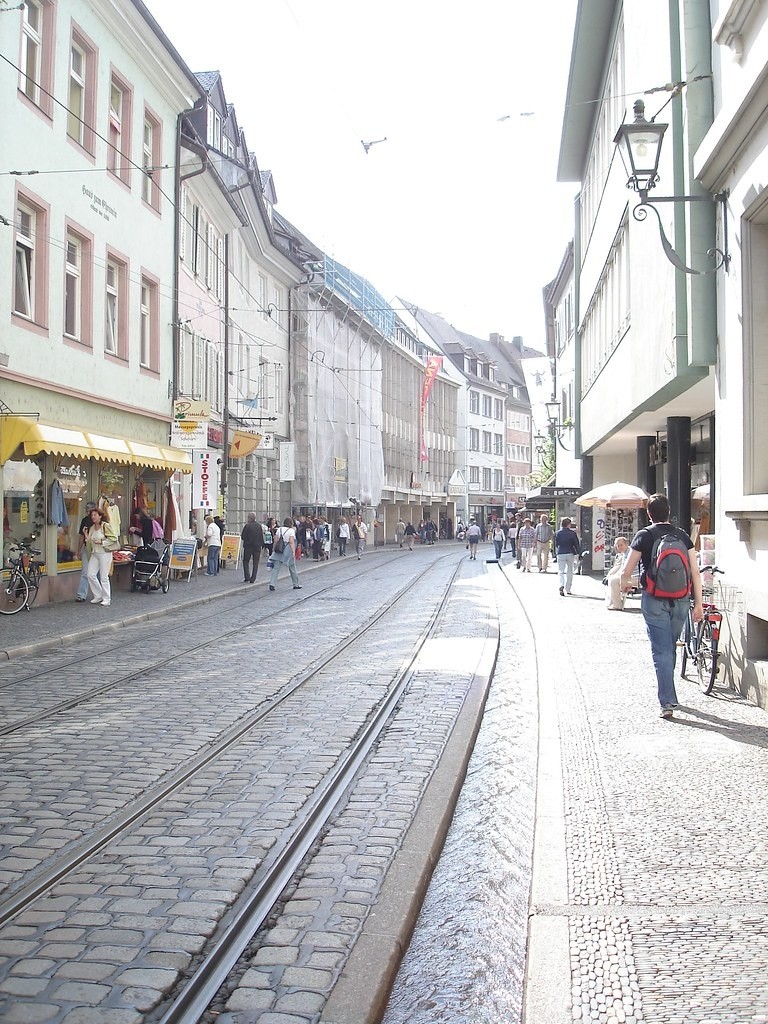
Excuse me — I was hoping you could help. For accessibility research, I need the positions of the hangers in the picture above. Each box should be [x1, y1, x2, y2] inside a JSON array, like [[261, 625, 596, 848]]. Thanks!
[[99, 494, 117, 506]]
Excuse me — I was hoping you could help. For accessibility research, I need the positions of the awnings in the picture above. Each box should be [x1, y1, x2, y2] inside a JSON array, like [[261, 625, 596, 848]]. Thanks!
[[0, 413, 193, 473]]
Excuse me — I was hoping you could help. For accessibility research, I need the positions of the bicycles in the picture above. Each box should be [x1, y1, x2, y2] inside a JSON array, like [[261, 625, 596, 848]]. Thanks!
[[0, 542, 44, 615], [675, 565, 725, 696]]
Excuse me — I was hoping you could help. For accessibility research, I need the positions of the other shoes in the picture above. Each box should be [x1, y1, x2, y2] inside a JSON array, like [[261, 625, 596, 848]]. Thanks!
[[527, 569, 530, 572], [75, 596, 85, 602], [270, 585, 275, 591], [660, 707, 673, 719], [539, 568, 542, 572], [244, 578, 250, 582], [609, 605, 622, 611], [293, 584, 302, 589], [320, 557, 325, 561], [343, 553, 346, 556], [313, 559, 318, 562], [522, 567, 526, 572], [559, 586, 565, 596]]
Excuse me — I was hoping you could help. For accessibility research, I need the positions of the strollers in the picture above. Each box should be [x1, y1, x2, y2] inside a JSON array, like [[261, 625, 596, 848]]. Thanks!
[[131, 537, 171, 594]]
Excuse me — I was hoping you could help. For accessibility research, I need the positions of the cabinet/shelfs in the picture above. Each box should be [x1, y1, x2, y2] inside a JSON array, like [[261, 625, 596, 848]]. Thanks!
[[603, 506, 635, 574]]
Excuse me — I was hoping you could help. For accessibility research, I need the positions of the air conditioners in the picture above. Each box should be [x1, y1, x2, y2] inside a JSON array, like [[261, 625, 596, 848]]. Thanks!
[[229, 447, 254, 473]]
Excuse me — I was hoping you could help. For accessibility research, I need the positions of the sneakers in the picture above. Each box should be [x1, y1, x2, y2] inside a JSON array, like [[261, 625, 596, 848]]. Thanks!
[[204, 571, 215, 576], [102, 599, 112, 605], [90, 597, 102, 604]]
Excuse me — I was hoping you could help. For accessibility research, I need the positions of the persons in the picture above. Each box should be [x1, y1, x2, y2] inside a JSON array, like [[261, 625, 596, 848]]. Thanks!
[[518, 518, 535, 572], [418, 518, 439, 545], [269, 517, 302, 590], [492, 521, 516, 559], [620, 494, 704, 718], [203, 516, 223, 576], [555, 519, 581, 596], [337, 517, 350, 556], [265, 517, 280, 556], [395, 518, 419, 550], [292, 515, 332, 562], [129, 507, 154, 546], [458, 518, 491, 560], [82, 508, 118, 605], [534, 515, 554, 572], [601, 536, 645, 611], [241, 512, 263, 583], [75, 502, 96, 599], [353, 515, 368, 560]]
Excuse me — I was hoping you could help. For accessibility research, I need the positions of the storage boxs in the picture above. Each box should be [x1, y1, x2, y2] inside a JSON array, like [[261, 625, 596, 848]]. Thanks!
[[698, 533, 714, 585]]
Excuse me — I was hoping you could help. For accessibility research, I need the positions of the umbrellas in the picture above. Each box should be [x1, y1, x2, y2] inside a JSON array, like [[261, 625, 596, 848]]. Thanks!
[[573, 481, 651, 538]]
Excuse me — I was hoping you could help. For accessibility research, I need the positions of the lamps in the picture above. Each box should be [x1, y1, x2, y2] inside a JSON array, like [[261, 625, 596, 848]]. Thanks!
[[613, 98, 727, 277], [545, 392, 573, 450], [534, 429, 555, 471]]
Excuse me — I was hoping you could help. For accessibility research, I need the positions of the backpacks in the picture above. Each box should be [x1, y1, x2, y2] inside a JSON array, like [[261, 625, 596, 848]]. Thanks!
[[646, 526, 692, 599], [275, 527, 292, 553], [148, 518, 163, 538]]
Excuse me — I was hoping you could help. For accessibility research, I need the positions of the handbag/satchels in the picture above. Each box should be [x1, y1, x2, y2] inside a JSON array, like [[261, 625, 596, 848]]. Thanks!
[[102, 536, 121, 551]]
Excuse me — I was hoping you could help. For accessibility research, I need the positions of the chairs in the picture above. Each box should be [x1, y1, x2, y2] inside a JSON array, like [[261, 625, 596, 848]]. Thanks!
[[620, 585, 643, 611]]
